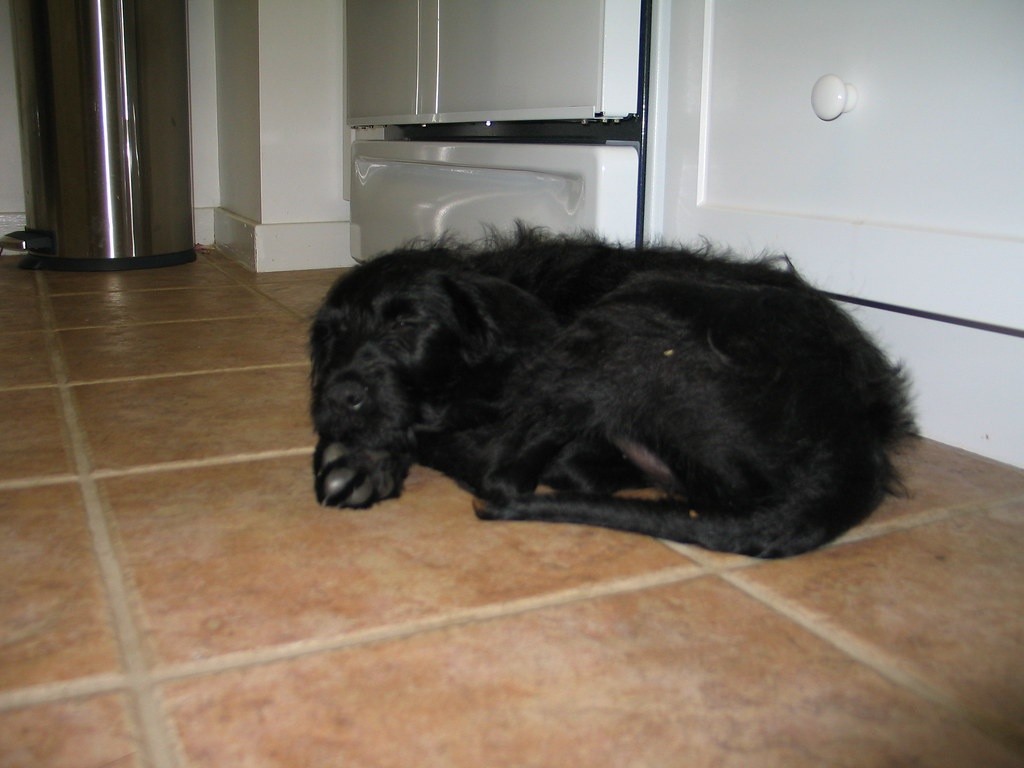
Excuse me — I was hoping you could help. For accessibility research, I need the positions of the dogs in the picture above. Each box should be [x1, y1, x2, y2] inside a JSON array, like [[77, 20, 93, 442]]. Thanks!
[[304, 238, 919, 560]]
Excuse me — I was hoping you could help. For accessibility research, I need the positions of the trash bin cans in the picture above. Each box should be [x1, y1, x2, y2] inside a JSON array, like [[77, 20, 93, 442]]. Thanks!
[[0, 0, 200, 273]]
